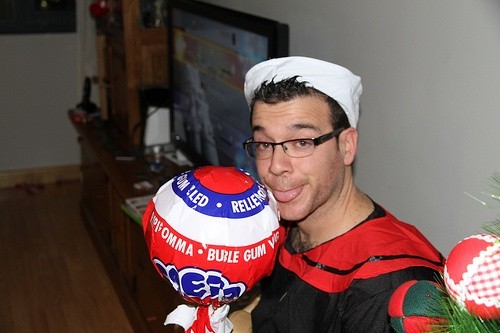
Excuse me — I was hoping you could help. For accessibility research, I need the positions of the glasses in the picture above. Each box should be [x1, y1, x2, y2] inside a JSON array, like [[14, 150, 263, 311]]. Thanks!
[[243, 129, 345, 158]]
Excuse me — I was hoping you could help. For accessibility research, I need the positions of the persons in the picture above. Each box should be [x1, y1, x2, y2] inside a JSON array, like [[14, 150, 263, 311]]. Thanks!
[[184, 56, 454, 332]]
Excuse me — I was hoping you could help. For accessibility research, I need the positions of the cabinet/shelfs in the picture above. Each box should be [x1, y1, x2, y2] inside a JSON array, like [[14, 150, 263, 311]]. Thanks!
[[68, 0, 197, 333]]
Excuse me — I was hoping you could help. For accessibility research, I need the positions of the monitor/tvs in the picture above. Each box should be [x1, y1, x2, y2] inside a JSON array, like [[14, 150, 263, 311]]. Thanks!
[[166, 0, 290, 169]]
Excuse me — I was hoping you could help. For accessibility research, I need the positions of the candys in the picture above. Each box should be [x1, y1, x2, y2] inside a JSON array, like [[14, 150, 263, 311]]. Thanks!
[[142, 166, 282, 333]]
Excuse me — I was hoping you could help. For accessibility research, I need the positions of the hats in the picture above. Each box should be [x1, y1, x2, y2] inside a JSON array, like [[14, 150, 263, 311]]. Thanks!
[[244, 54, 362, 128]]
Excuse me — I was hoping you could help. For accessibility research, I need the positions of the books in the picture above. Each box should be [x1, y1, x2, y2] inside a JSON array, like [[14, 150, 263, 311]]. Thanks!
[[118, 194, 154, 227]]
